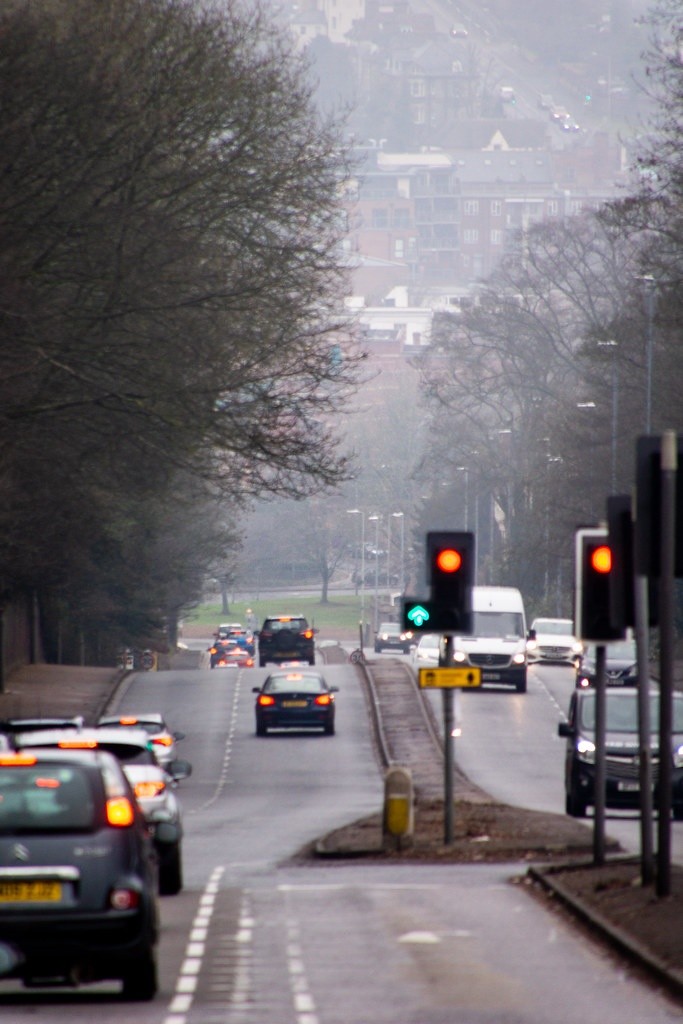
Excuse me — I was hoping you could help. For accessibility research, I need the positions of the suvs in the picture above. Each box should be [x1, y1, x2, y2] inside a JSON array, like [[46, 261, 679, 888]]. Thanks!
[[252, 614, 318, 667]]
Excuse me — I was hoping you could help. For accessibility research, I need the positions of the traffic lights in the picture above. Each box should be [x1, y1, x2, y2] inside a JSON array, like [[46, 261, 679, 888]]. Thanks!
[[399, 531, 474, 635], [572, 526, 626, 642], [584, 93, 592, 103]]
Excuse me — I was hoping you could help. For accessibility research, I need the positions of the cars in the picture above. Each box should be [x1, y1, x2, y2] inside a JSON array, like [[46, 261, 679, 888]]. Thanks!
[[252, 671, 339, 737], [374, 623, 415, 655], [558, 685, 683, 821], [538, 93, 580, 135], [575, 637, 640, 689], [416, 633, 442, 660], [0, 714, 194, 1003], [448, 23, 469, 37], [499, 85, 517, 103], [527, 618, 584, 667], [207, 624, 258, 670]]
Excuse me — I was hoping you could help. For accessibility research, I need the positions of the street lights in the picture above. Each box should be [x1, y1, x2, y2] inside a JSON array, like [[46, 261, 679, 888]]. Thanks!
[[633, 274, 656, 434], [455, 430, 516, 587], [368, 515, 378, 624], [535, 436, 567, 616], [346, 510, 365, 631], [576, 403, 598, 525], [440, 467, 470, 532], [391, 512, 405, 595], [597, 341, 618, 494]]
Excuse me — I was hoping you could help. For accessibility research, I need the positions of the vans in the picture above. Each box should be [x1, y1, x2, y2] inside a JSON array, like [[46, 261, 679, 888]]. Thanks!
[[439, 587, 537, 693]]
